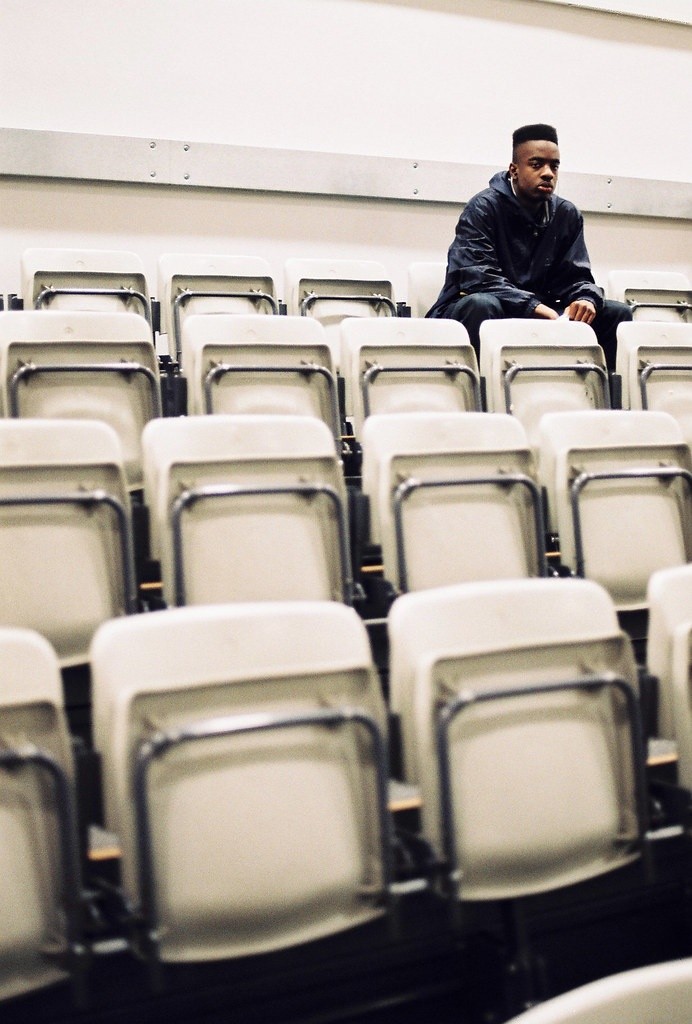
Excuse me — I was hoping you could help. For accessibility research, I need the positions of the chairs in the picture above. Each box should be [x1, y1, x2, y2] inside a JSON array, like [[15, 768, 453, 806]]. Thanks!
[[350, 411, 558, 612], [8, 248, 159, 348], [0, 310, 175, 494], [402, 262, 448, 318], [505, 958, 692, 1024], [87, 601, 399, 968], [645, 563, 692, 792], [0, 626, 87, 998], [387, 578, 650, 902], [140, 414, 371, 618], [157, 254, 284, 389], [178, 315, 353, 463], [0, 417, 147, 670], [282, 260, 407, 373], [609, 269, 692, 324], [614, 320, 692, 453], [341, 316, 489, 449], [536, 408, 692, 612], [478, 318, 615, 458]]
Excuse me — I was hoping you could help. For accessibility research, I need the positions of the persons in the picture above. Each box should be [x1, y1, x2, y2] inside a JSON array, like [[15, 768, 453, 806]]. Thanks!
[[424, 124, 633, 375]]
